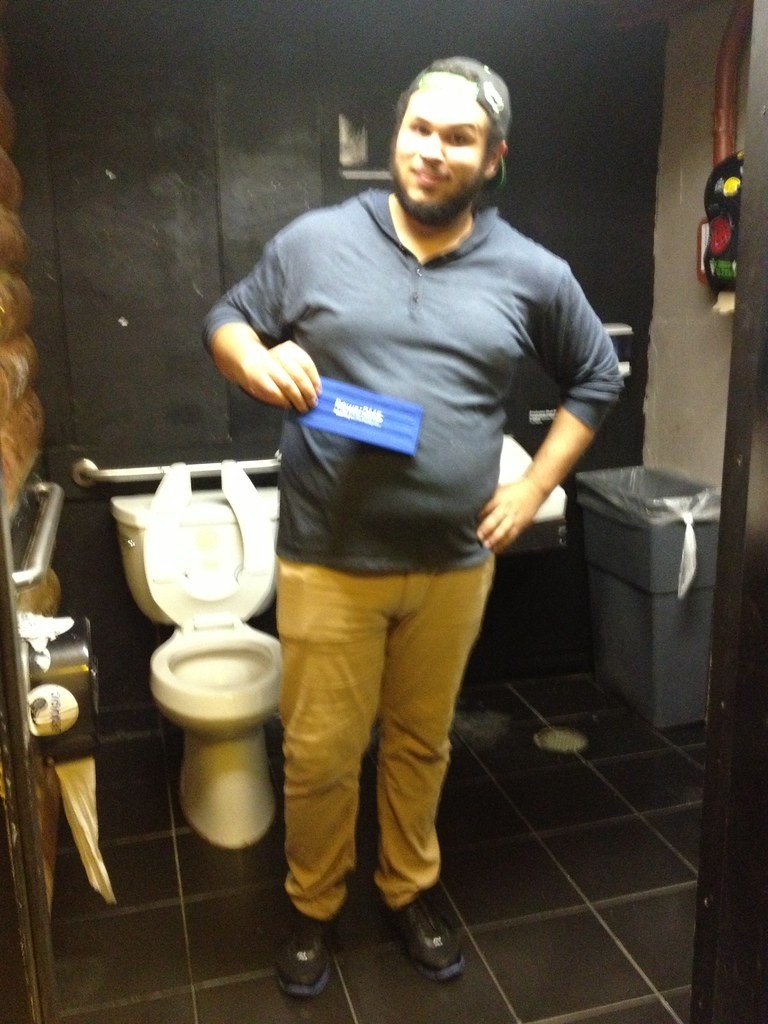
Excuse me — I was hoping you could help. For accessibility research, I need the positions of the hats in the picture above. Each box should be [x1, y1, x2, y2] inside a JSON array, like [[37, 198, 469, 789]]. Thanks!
[[408, 55, 511, 196]]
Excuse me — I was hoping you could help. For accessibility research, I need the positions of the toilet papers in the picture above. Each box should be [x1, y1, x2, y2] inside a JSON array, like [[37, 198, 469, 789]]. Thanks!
[[25, 614, 116, 907]]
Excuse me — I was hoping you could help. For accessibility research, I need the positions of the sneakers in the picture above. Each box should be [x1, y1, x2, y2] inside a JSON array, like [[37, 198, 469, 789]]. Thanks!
[[275, 909, 332, 997], [400, 893, 466, 981]]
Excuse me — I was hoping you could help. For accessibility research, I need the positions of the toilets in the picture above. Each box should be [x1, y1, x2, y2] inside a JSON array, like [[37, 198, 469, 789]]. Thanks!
[[110, 455, 282, 848]]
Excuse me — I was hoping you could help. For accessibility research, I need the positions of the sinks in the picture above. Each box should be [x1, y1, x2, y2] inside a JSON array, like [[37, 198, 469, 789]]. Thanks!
[[493, 433, 566, 557]]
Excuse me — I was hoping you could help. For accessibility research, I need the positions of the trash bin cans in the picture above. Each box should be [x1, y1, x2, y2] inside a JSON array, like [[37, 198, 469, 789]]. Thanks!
[[575, 466, 722, 730]]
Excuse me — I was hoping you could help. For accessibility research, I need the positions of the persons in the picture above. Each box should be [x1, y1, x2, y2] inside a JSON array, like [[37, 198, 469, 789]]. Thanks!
[[202, 56, 625, 996]]
